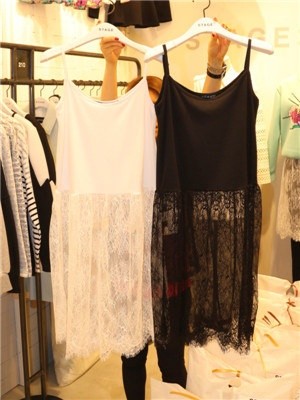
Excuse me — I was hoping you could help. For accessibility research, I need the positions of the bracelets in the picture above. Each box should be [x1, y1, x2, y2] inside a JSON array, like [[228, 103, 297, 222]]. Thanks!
[[207, 62, 228, 74]]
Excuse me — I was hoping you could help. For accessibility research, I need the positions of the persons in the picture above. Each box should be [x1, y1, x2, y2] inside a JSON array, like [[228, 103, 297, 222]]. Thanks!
[[100, 17, 236, 400]]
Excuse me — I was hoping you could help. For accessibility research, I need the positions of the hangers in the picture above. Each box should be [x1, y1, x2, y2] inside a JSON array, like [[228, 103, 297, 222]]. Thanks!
[[0, 76, 126, 118], [39, 0, 167, 63], [143, 0, 273, 61]]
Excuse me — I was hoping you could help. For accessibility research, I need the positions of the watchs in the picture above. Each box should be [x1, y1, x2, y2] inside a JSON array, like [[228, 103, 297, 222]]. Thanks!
[[207, 69, 226, 79]]
